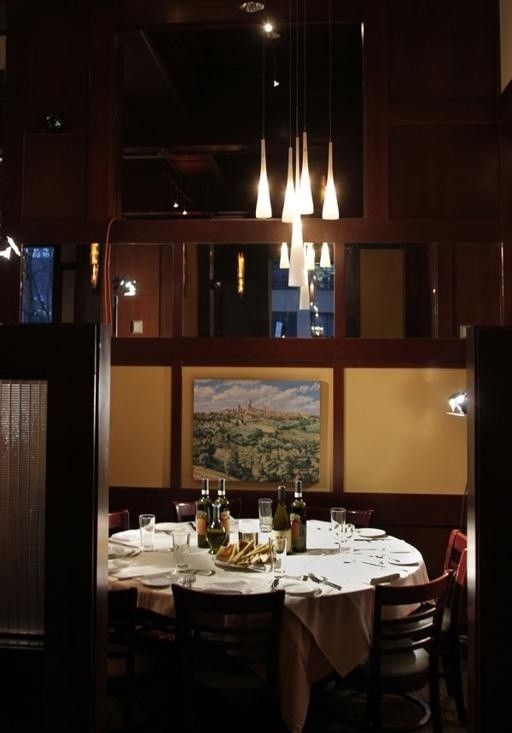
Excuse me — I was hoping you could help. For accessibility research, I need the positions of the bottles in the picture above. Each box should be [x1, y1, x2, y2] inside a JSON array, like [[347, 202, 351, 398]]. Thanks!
[[195, 476, 234, 555], [272, 479, 308, 555]]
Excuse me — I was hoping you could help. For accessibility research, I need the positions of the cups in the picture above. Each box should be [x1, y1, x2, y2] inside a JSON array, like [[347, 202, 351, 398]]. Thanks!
[[137, 497, 358, 573]]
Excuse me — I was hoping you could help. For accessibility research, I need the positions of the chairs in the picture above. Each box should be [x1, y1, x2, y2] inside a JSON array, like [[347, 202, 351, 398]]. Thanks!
[[172, 501, 195, 522], [108, 510, 129, 533], [172, 584, 286, 733], [347, 509, 374, 528], [429, 529, 467, 728], [106, 587, 138, 733], [368, 570, 454, 733]]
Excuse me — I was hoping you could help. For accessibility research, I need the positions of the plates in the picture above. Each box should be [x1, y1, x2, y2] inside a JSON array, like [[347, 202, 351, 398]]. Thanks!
[[109, 520, 420, 597]]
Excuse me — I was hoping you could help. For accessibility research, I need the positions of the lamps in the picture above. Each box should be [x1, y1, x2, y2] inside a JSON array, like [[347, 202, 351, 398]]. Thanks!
[[446, 391, 467, 417], [114, 274, 137, 298], [254, 0, 341, 310]]
